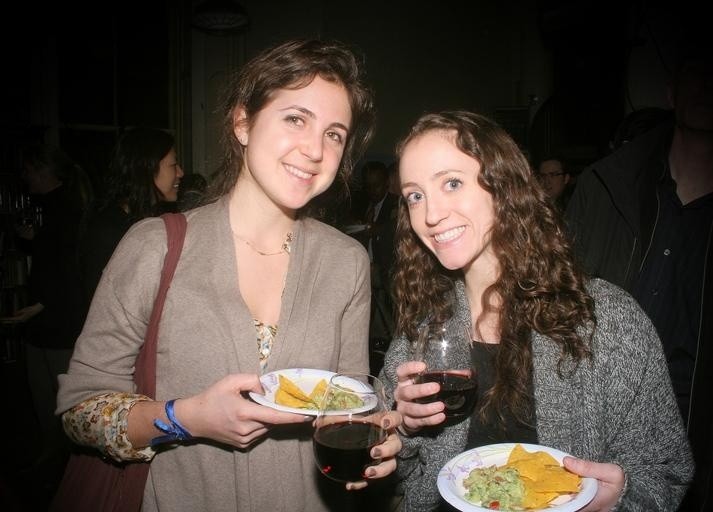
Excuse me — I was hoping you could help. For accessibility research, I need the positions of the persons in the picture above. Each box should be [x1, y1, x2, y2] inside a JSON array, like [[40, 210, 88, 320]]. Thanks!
[[373, 110, 696, 510], [55, 44, 403, 510], [606, 107, 677, 155], [305, 160, 402, 338], [533, 137, 574, 225], [13, 119, 206, 511], [565, 63, 712, 510]]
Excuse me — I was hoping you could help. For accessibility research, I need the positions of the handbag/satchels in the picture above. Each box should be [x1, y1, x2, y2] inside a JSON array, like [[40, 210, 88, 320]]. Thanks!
[[50, 212, 187, 512]]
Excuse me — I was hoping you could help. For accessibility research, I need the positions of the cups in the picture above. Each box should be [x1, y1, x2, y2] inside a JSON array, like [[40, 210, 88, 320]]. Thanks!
[[313, 373, 387, 485], [414, 321, 481, 422]]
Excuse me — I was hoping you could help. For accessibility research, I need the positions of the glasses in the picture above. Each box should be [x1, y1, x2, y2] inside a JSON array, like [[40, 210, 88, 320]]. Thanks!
[[541, 171, 564, 178]]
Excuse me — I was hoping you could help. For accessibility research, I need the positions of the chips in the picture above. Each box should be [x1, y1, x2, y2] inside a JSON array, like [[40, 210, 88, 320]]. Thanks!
[[275, 374, 329, 408], [496, 444, 583, 510]]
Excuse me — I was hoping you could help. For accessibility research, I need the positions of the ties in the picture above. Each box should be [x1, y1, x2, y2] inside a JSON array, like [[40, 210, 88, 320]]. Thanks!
[[366, 202, 377, 223]]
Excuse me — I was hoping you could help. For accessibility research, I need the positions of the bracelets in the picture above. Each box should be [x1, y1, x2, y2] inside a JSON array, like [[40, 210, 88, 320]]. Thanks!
[[152, 399, 193, 445]]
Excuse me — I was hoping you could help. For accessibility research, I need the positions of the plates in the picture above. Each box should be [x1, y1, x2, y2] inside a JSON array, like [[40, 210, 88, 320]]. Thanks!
[[249, 366, 379, 417], [436, 442, 597, 511]]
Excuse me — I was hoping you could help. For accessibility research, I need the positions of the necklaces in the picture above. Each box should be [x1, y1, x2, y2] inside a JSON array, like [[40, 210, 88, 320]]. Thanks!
[[233, 230, 293, 256]]
